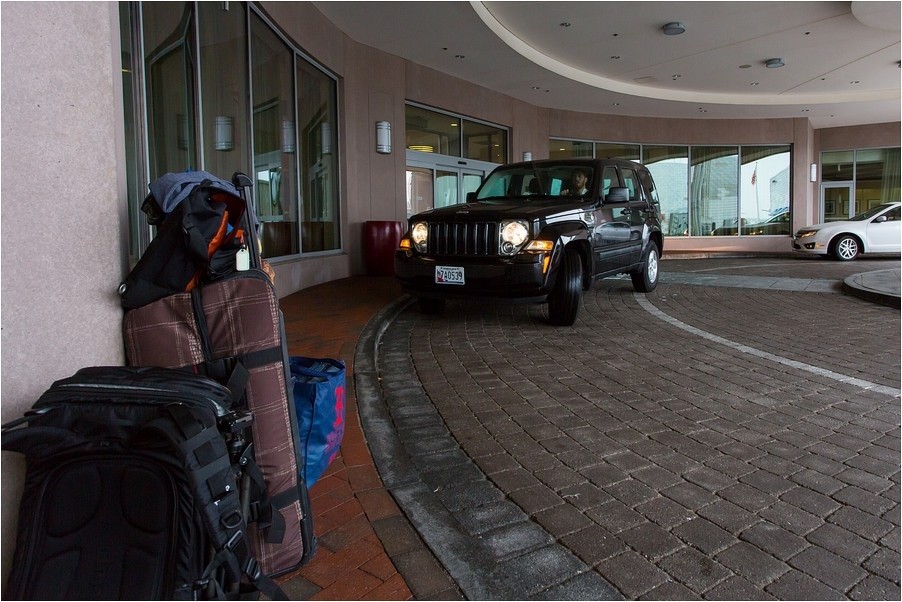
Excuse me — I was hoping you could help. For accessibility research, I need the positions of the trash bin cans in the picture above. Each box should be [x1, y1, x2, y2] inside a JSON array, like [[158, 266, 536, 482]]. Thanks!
[[364, 220, 403, 275]]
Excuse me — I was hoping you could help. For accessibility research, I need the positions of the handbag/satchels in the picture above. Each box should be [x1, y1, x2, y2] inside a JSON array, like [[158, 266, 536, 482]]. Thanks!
[[290, 355, 344, 492]]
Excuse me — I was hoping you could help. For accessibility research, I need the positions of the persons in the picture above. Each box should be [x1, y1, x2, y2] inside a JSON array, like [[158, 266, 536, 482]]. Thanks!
[[560, 169, 589, 196]]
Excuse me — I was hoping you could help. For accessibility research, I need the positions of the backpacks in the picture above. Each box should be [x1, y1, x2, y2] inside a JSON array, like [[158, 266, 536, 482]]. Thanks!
[[1, 366, 263, 601], [119, 179, 246, 307]]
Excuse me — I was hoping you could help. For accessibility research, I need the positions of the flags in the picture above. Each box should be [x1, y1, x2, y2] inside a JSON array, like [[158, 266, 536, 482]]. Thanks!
[[751, 169, 756, 184]]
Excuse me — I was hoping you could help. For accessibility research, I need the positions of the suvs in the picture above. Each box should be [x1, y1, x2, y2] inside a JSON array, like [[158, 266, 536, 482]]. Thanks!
[[393, 157, 665, 326]]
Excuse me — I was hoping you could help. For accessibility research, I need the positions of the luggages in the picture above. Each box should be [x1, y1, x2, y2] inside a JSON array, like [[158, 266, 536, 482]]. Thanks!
[[127, 173, 316, 579]]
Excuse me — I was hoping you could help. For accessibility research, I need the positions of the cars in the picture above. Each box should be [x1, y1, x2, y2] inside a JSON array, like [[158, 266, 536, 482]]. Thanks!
[[791, 201, 901, 262], [714, 209, 790, 236]]
